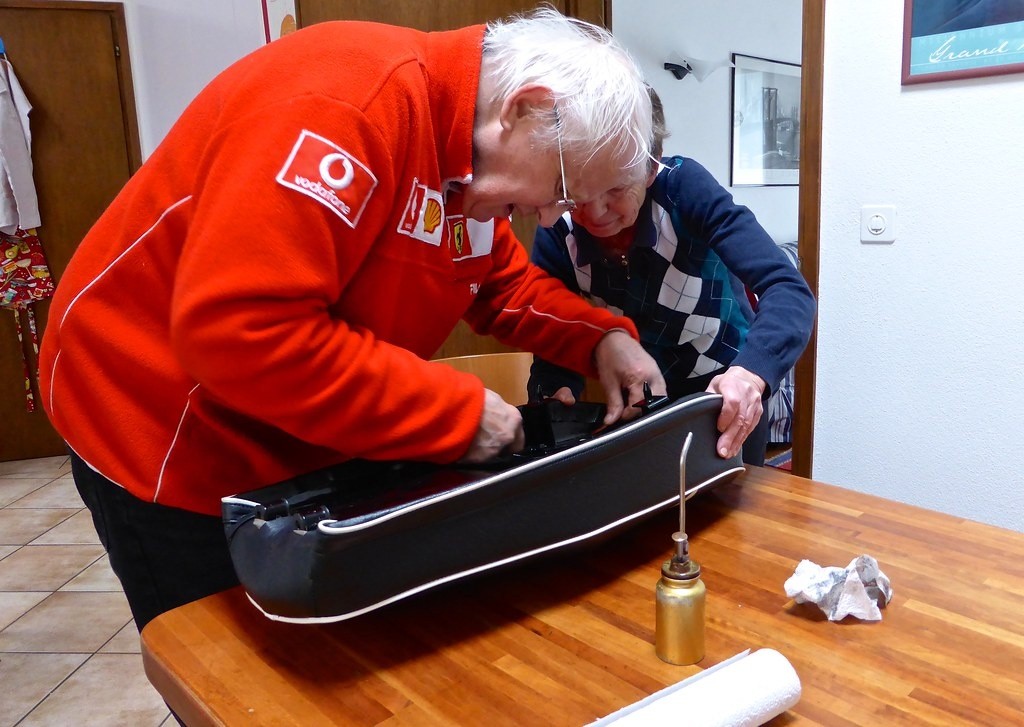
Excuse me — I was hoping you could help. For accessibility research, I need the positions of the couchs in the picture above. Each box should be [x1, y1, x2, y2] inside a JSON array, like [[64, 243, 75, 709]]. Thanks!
[[739, 240, 798, 444]]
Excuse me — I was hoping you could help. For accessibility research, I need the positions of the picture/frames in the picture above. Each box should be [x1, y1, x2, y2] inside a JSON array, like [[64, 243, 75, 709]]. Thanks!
[[901, 0, 1024, 85], [730, 52, 802, 188]]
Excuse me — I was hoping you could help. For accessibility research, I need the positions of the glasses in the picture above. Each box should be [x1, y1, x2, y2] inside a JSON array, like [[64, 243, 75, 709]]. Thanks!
[[554, 102, 578, 212]]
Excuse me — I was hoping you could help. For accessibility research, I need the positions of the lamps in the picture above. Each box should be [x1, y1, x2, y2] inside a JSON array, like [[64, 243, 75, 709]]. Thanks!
[[664, 56, 735, 83]]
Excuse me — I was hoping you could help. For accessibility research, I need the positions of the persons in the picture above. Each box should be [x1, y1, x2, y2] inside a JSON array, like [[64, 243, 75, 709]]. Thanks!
[[36, 7, 668, 638], [527, 80, 817, 467]]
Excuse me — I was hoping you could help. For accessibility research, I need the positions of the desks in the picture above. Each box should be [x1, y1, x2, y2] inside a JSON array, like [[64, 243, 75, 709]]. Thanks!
[[140, 462, 1024, 727]]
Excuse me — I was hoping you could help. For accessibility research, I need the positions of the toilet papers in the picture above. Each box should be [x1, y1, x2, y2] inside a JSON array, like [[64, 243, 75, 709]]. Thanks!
[[581, 646, 805, 726]]
